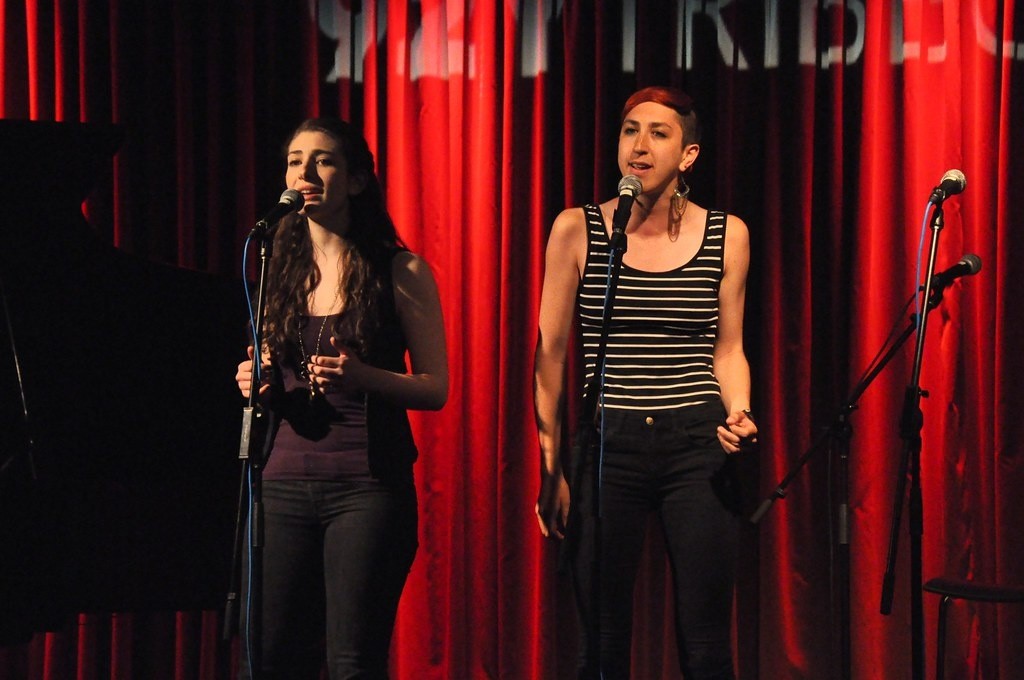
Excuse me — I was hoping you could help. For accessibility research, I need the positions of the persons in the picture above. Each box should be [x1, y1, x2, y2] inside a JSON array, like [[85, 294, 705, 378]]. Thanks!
[[235, 117, 450, 680], [534, 85, 759, 680]]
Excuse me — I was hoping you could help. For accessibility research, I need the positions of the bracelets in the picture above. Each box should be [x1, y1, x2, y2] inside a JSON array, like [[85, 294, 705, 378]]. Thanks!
[[741, 408, 755, 422]]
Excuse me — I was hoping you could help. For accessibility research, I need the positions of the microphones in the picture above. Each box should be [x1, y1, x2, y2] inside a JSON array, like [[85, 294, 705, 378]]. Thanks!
[[609, 174, 644, 252], [249, 189, 305, 239], [929, 168, 967, 204], [920, 254, 983, 292]]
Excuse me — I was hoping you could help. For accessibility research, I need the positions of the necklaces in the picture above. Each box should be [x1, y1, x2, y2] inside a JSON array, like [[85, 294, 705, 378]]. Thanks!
[[298, 289, 339, 401]]
